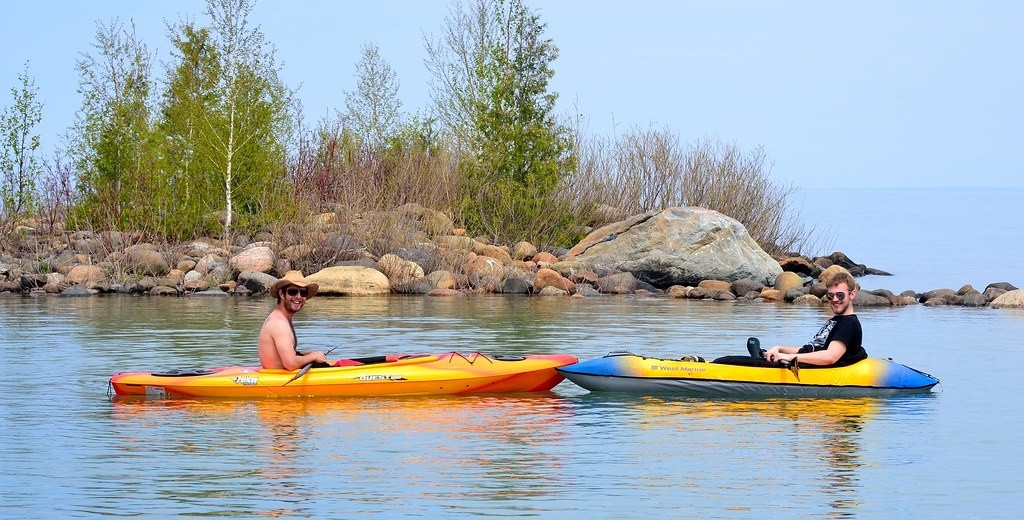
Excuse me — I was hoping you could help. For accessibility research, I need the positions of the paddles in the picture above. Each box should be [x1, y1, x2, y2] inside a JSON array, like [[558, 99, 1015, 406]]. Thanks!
[[282, 345, 338, 387], [747, 337, 801, 382]]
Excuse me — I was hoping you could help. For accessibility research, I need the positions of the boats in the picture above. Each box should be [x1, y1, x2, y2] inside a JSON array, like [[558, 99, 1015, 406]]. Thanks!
[[555, 351, 941, 401], [106, 351, 578, 396]]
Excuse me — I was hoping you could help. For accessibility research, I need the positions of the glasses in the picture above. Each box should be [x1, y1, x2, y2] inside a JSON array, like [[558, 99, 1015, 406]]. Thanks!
[[283, 289, 308, 297], [827, 291, 852, 300]]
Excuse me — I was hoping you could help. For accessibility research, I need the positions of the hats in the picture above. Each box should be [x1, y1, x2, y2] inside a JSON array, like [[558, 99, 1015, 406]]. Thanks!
[[270, 270, 320, 301]]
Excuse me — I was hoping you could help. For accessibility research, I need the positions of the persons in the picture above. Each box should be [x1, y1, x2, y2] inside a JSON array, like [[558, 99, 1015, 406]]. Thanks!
[[259, 270, 331, 369], [695, 272, 862, 368]]
